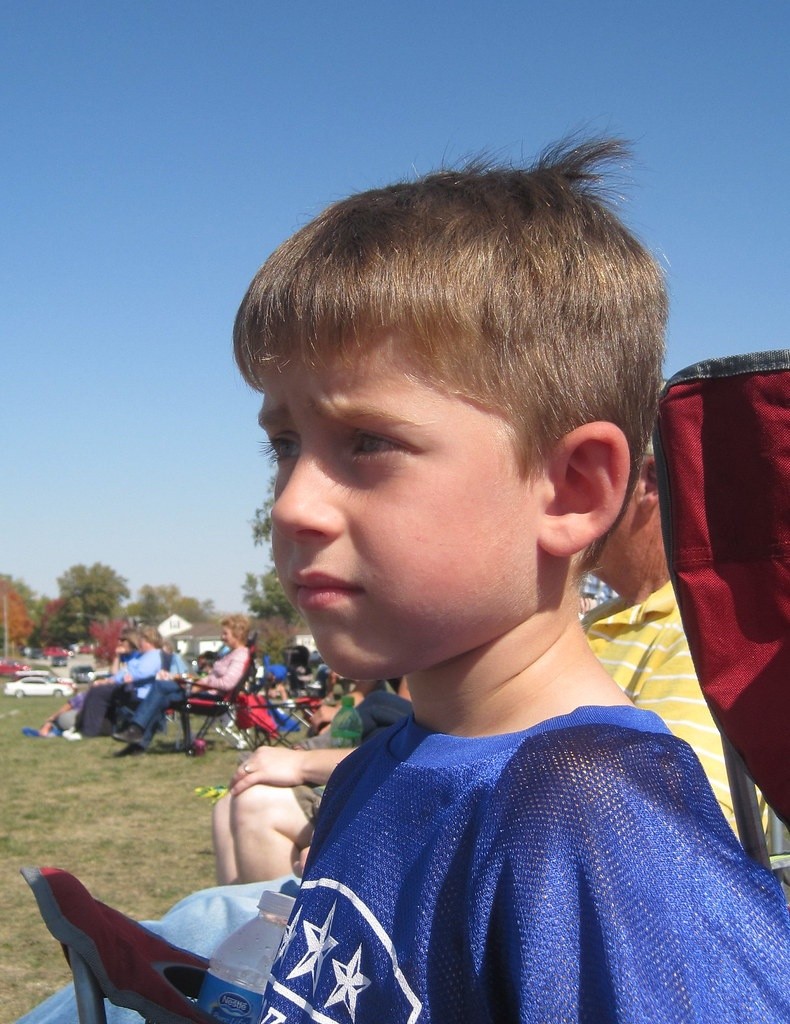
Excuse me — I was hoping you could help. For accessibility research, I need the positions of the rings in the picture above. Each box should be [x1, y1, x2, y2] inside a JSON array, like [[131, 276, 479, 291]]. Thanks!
[[244, 764, 251, 773]]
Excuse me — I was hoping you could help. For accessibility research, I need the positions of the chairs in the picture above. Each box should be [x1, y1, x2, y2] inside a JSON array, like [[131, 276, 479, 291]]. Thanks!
[[20, 346, 790, 1024]]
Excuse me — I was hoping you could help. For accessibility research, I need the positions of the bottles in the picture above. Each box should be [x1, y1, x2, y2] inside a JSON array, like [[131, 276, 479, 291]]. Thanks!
[[195, 735, 205, 756], [330, 696, 363, 749]]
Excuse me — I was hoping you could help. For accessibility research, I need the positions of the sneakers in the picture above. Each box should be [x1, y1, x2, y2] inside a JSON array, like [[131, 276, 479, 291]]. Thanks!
[[114, 744, 146, 759], [112, 724, 144, 745]]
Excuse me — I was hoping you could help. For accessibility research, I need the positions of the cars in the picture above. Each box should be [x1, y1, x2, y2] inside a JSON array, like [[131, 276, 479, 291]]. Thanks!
[[4, 677, 73, 698], [73, 665, 96, 683], [0, 658, 29, 674], [18, 643, 90, 667]]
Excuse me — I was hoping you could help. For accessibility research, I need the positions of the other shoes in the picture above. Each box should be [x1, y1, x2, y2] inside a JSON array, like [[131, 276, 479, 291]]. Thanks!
[[63, 726, 81, 742], [51, 728, 64, 735], [22, 727, 42, 737]]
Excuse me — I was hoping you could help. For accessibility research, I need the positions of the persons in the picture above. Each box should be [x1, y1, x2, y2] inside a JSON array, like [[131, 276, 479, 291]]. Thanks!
[[22, 624, 188, 736], [211, 441, 772, 887], [112, 613, 250, 755], [261, 664, 288, 700], [232, 133, 790, 1024]]
